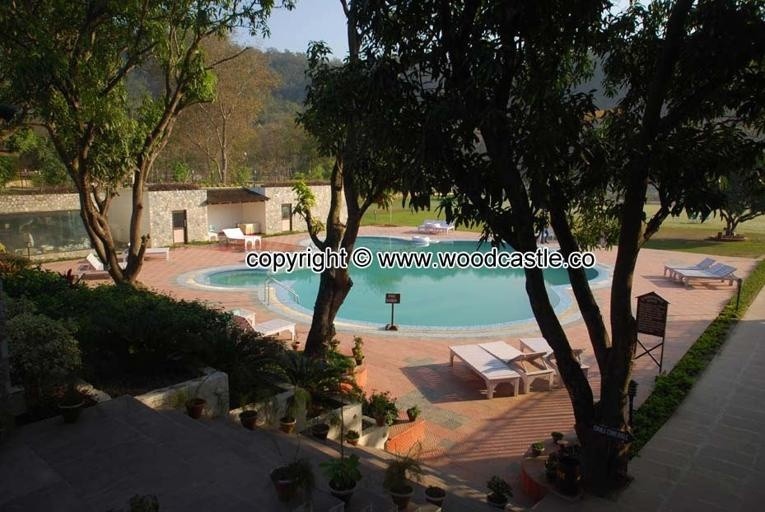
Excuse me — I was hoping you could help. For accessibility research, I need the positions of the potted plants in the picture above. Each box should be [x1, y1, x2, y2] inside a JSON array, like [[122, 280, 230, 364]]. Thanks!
[[424, 485, 448, 506], [545, 463, 557, 480], [312, 422, 330, 440], [319, 453, 362, 503], [370, 392, 420, 427], [239, 410, 258, 430], [486, 475, 514, 511], [382, 441, 423, 510], [270, 459, 314, 503], [186, 397, 207, 418], [551, 431, 564, 441], [346, 430, 359, 446], [532, 442, 545, 456], [278, 414, 296, 434], [57, 385, 86, 423]]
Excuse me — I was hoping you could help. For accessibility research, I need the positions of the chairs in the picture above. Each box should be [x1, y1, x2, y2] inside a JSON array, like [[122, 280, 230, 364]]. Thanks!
[[232, 228, 261, 249], [73, 248, 169, 277], [417, 219, 455, 236], [256, 318, 296, 342], [448, 337, 585, 400], [222, 228, 254, 252], [664, 257, 741, 289]]
